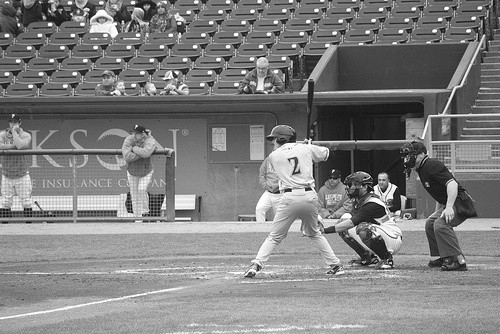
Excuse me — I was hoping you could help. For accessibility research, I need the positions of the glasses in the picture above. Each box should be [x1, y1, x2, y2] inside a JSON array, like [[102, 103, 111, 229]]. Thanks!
[[329, 177, 341, 179]]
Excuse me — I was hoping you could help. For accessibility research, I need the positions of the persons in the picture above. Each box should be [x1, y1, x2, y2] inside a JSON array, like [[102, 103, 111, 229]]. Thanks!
[[318, 169, 403, 269], [255, 157, 280, 222], [0, 113, 32, 223], [0, 0, 178, 34], [122, 123, 156, 223], [238, 57, 285, 94], [400, 142, 475, 272], [95, 70, 189, 95], [242, 125, 341, 277]]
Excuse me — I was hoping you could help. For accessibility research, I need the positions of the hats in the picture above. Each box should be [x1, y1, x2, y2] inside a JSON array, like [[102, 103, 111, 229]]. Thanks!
[[129, 125, 145, 132], [327, 169, 341, 178], [8, 114, 22, 122], [162, 71, 178, 80], [102, 70, 116, 77], [411, 141, 427, 155]]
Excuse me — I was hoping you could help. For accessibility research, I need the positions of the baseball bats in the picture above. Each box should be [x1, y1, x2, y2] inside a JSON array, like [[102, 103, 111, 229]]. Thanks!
[[34, 201, 44, 214], [307, 78, 314, 139]]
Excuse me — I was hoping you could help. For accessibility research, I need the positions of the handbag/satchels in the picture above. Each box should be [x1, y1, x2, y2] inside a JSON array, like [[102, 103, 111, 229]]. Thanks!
[[454, 189, 478, 217]]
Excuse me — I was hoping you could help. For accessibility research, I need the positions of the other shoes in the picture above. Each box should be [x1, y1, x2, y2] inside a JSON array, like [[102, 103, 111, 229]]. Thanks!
[[429, 257, 451, 267], [441, 259, 467, 271], [348, 255, 380, 267], [325, 266, 345, 276], [377, 259, 394, 268], [244, 264, 261, 277]]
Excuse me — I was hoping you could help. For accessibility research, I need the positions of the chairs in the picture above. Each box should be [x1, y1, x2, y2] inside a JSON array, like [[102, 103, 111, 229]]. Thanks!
[[0, 0, 495, 98]]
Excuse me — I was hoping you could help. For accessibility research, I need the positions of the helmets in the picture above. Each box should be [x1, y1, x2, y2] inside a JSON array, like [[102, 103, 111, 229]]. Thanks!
[[344, 171, 374, 191], [266, 124, 296, 144]]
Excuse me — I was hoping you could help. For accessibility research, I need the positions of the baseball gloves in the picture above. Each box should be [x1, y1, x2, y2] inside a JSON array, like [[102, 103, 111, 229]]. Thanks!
[[301, 214, 324, 234]]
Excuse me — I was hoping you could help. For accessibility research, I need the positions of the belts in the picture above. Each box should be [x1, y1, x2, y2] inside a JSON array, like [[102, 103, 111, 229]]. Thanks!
[[284, 187, 315, 192], [268, 189, 280, 194]]
